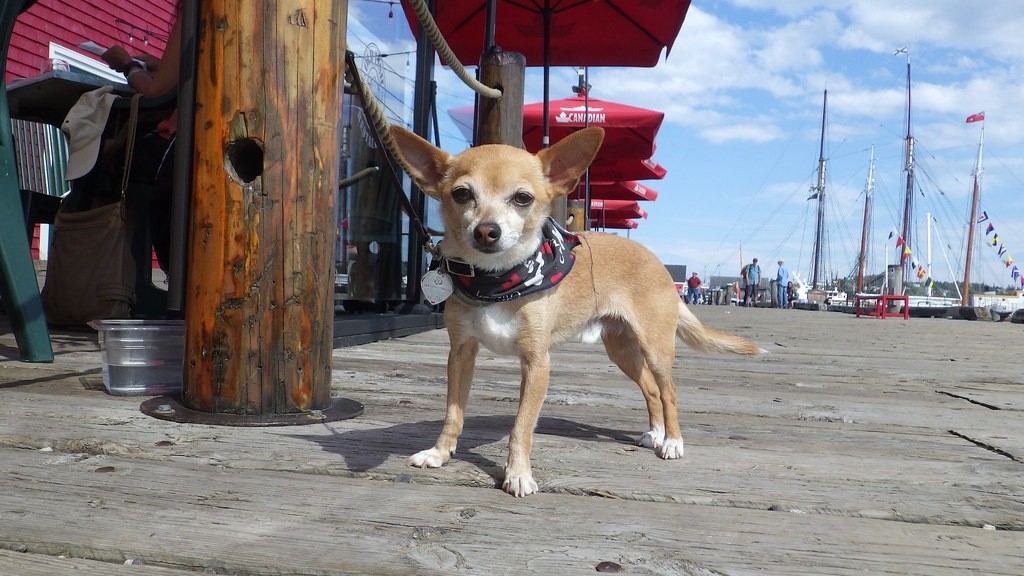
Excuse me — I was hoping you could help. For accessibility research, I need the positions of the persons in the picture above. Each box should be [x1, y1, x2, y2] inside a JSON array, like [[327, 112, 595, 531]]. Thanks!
[[741, 257, 760, 309], [89, 0, 182, 211], [687, 271, 701, 305], [776, 259, 800, 308]]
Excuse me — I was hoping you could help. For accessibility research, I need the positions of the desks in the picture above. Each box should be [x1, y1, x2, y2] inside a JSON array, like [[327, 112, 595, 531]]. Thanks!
[[4, 71, 144, 245]]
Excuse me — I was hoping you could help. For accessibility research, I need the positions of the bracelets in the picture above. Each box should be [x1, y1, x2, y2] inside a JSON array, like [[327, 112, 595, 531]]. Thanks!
[[124, 61, 145, 81]]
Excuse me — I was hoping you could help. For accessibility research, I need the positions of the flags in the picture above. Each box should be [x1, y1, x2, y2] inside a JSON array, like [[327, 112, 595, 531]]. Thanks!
[[893, 47, 908, 58], [975, 210, 1024, 291], [966, 110, 985, 124], [888, 226, 930, 287]]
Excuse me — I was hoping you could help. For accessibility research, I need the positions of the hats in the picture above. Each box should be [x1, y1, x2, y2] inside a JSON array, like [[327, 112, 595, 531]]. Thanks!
[[61, 86, 122, 181]]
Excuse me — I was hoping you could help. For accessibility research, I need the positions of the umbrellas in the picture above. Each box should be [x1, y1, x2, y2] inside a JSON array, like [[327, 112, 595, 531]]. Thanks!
[[401, 0, 691, 231]]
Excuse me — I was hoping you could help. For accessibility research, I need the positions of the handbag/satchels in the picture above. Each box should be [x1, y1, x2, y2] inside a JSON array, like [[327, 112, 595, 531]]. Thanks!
[[41, 93, 140, 325]]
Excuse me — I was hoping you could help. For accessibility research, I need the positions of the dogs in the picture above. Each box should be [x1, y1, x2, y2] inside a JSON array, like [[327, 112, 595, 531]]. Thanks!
[[388, 123, 769, 498]]
